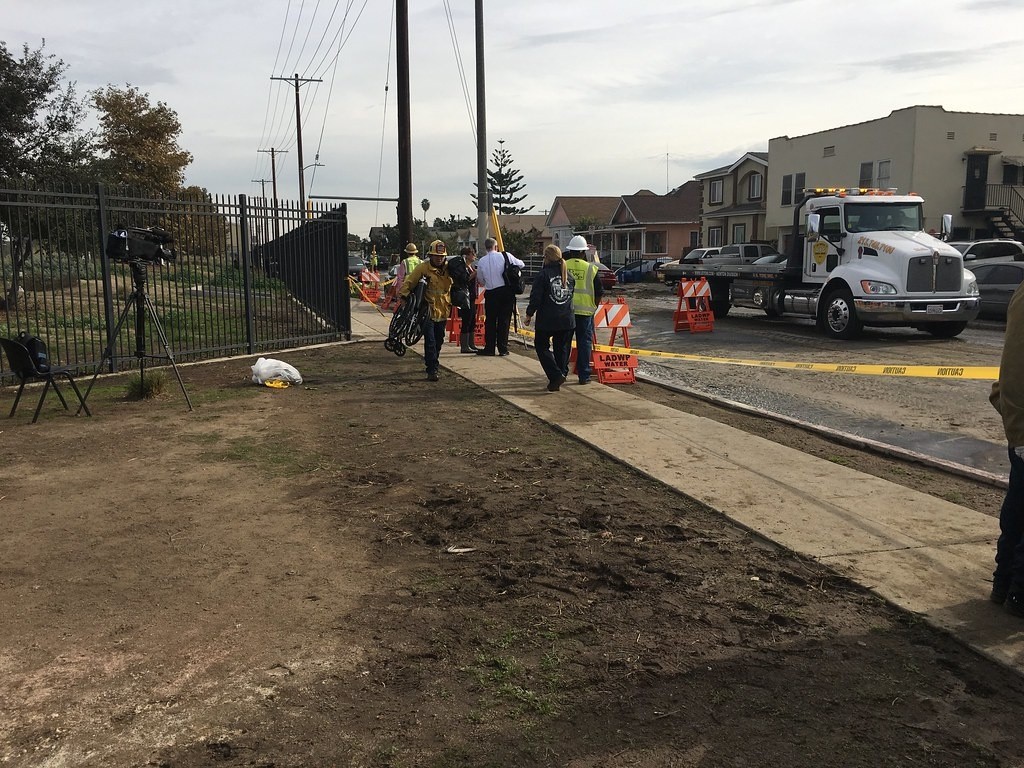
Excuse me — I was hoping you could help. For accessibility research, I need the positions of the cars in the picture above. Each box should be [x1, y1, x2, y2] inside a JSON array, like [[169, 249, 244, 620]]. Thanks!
[[965, 261, 1024, 313], [659, 260, 680, 269], [368, 254, 390, 269], [750, 254, 790, 265], [348, 257, 368, 272], [589, 263, 616, 290]]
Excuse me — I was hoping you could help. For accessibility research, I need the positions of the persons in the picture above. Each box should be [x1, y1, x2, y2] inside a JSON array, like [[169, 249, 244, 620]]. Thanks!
[[477, 237, 525, 357], [564, 235, 603, 384], [396, 242, 423, 296], [457, 246, 481, 353], [370, 251, 378, 272], [989, 278, 1024, 617], [524, 245, 577, 391], [397, 239, 454, 381]]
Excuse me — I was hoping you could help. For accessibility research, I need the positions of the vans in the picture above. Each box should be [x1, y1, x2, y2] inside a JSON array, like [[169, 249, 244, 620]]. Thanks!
[[683, 248, 722, 264]]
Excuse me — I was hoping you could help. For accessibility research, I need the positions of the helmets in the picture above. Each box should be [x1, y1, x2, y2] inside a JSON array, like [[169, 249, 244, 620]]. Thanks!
[[565, 235, 590, 250], [426, 240, 447, 256], [404, 243, 418, 253]]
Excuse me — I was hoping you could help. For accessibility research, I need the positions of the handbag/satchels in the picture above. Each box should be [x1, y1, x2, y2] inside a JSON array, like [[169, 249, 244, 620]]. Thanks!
[[11, 331, 51, 373], [251, 357, 303, 386]]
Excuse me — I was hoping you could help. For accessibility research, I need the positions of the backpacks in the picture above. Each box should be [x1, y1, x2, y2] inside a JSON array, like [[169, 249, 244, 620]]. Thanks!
[[448, 257, 471, 312], [501, 251, 525, 294]]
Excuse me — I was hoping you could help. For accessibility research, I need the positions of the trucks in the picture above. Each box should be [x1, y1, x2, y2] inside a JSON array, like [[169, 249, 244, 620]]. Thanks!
[[655, 188, 982, 341]]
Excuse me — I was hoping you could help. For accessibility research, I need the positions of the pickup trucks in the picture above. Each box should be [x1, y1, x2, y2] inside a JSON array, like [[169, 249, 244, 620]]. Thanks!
[[699, 244, 780, 265]]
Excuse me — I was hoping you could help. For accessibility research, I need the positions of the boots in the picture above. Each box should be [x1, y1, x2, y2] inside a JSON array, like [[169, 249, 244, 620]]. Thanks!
[[469, 332, 480, 350], [460, 333, 478, 353]]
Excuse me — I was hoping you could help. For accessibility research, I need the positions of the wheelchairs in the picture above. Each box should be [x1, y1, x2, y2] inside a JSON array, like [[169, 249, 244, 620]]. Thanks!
[[383, 275, 431, 356]]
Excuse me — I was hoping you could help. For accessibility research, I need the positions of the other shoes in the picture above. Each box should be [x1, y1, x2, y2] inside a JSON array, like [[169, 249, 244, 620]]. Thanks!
[[988, 577, 1009, 604], [547, 375, 566, 391], [578, 377, 591, 385], [428, 373, 439, 382], [1003, 584, 1024, 618], [476, 350, 495, 356], [499, 351, 510, 356]]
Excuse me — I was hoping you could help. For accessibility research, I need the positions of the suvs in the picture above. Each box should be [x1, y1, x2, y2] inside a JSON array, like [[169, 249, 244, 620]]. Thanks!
[[946, 238, 1024, 269]]
